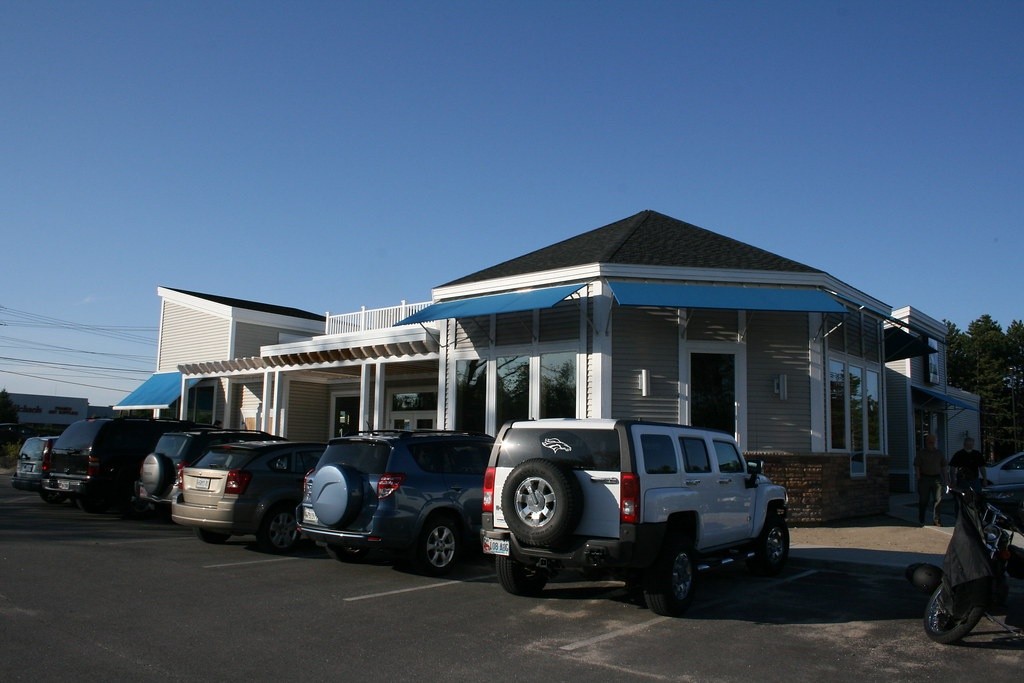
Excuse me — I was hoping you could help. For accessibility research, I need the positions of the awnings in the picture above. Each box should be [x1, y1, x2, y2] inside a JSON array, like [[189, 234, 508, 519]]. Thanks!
[[608, 281, 850, 314], [393, 283, 589, 327], [910, 383, 982, 413], [113, 372, 202, 410], [884, 327, 939, 364], [823, 287, 950, 348]]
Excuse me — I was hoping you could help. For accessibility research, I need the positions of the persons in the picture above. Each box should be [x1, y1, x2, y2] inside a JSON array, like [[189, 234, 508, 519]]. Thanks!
[[949, 436, 988, 525], [912, 432, 948, 528]]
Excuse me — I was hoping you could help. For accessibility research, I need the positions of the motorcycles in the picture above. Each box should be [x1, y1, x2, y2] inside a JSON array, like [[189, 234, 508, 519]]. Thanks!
[[924, 467, 1024, 645]]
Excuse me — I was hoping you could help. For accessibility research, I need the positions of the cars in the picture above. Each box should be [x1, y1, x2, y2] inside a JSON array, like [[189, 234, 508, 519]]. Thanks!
[[977, 450, 1024, 487]]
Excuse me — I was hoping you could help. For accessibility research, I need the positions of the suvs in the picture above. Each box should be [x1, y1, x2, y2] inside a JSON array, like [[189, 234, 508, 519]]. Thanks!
[[12, 435, 61, 493], [172, 439, 328, 555], [131, 428, 291, 525], [480, 417, 790, 615], [295, 429, 497, 578], [39, 417, 224, 516]]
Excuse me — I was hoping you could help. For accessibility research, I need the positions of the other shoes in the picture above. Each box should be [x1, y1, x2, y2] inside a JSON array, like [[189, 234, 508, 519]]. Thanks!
[[918, 522, 925, 528], [934, 521, 942, 527]]
